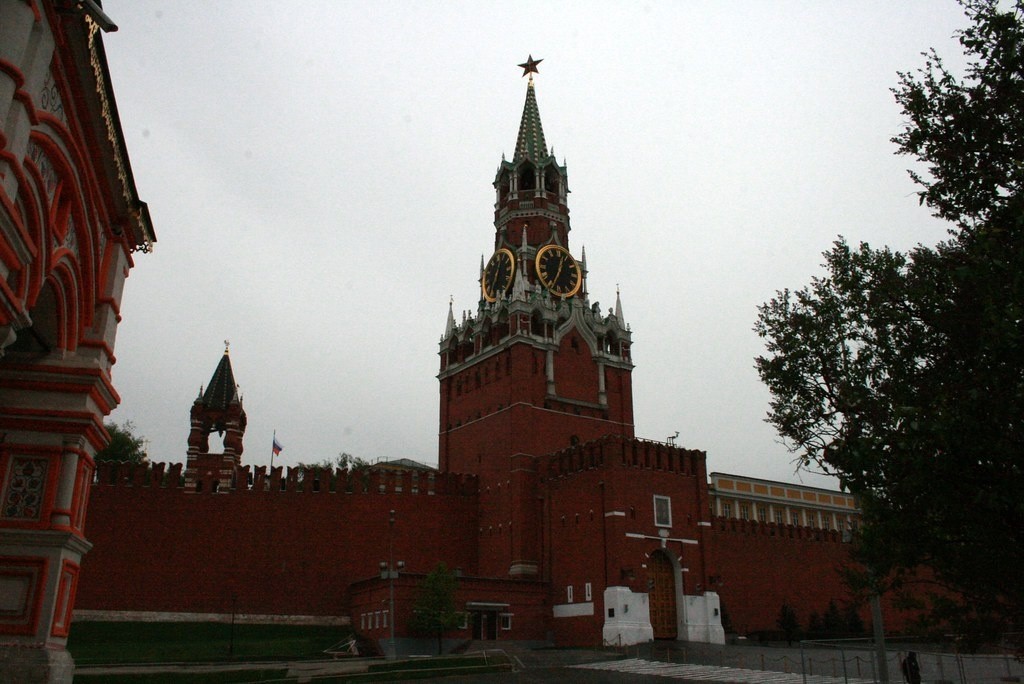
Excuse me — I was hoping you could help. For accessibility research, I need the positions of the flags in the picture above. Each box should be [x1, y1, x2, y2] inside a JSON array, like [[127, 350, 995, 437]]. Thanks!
[[273, 435, 282, 457]]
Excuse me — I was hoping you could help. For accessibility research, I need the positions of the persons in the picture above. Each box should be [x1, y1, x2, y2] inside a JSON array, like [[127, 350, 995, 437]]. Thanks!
[[902, 651, 921, 684]]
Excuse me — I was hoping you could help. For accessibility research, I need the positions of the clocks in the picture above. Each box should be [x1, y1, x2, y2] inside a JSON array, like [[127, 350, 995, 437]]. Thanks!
[[535, 245, 582, 297], [482, 248, 514, 302]]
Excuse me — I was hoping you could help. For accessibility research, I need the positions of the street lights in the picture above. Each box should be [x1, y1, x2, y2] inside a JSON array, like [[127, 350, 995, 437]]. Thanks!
[[376, 508, 406, 661]]
